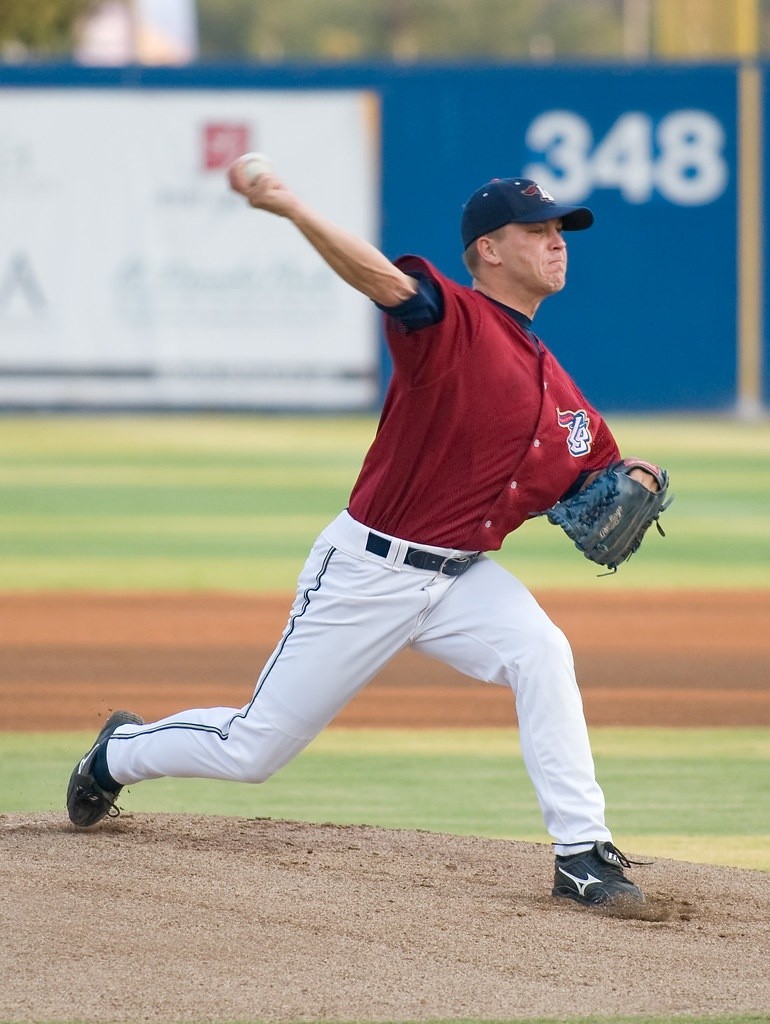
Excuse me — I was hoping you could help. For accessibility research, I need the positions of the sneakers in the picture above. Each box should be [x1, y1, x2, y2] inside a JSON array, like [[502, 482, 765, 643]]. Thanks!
[[552, 840, 654, 909], [67, 710, 144, 827]]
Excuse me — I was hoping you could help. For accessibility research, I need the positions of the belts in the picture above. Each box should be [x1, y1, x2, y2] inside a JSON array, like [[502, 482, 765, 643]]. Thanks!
[[365, 532, 482, 578]]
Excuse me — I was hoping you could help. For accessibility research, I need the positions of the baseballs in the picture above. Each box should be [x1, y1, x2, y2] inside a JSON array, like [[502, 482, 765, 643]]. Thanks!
[[233, 150, 278, 191]]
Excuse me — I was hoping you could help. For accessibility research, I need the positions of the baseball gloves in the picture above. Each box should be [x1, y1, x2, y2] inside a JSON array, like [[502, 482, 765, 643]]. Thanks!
[[542, 456, 672, 572]]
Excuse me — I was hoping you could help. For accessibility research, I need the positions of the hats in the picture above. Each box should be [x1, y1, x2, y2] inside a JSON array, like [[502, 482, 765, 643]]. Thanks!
[[462, 178, 594, 251]]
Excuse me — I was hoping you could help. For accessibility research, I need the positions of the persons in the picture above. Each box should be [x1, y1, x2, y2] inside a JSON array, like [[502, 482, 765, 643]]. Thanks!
[[65, 165, 669, 922]]
